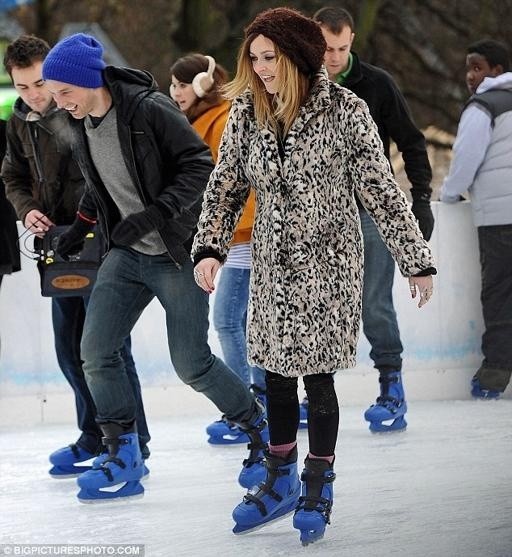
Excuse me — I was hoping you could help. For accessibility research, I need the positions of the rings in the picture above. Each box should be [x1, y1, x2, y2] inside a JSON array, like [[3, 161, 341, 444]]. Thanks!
[[409, 285, 416, 291], [193, 269, 201, 281], [420, 287, 431, 301]]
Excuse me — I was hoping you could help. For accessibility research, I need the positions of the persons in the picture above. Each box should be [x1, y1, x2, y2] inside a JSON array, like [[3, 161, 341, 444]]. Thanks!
[[166, 51, 269, 439], [299, 6, 435, 425], [2, 34, 153, 468], [189, 6, 439, 534], [438, 37, 512, 399], [41, 33, 270, 488]]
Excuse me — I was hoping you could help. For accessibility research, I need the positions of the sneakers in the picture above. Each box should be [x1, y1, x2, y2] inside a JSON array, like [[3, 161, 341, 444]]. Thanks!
[[472, 363, 510, 390], [299, 394, 310, 419]]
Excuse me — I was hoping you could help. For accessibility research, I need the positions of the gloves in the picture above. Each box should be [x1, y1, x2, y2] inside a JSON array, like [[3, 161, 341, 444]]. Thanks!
[[407, 187, 435, 242], [54, 212, 98, 262], [109, 202, 165, 247]]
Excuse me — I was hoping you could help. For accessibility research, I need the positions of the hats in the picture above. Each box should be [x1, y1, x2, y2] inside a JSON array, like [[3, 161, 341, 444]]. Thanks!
[[41, 32, 107, 88], [245, 6, 327, 79]]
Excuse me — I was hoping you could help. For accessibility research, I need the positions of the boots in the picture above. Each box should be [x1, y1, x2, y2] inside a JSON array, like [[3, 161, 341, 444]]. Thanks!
[[74, 418, 145, 491], [292, 451, 337, 530], [364, 371, 408, 422], [209, 417, 270, 445], [47, 441, 98, 467], [237, 412, 274, 488], [205, 382, 268, 435], [232, 444, 302, 527]]
